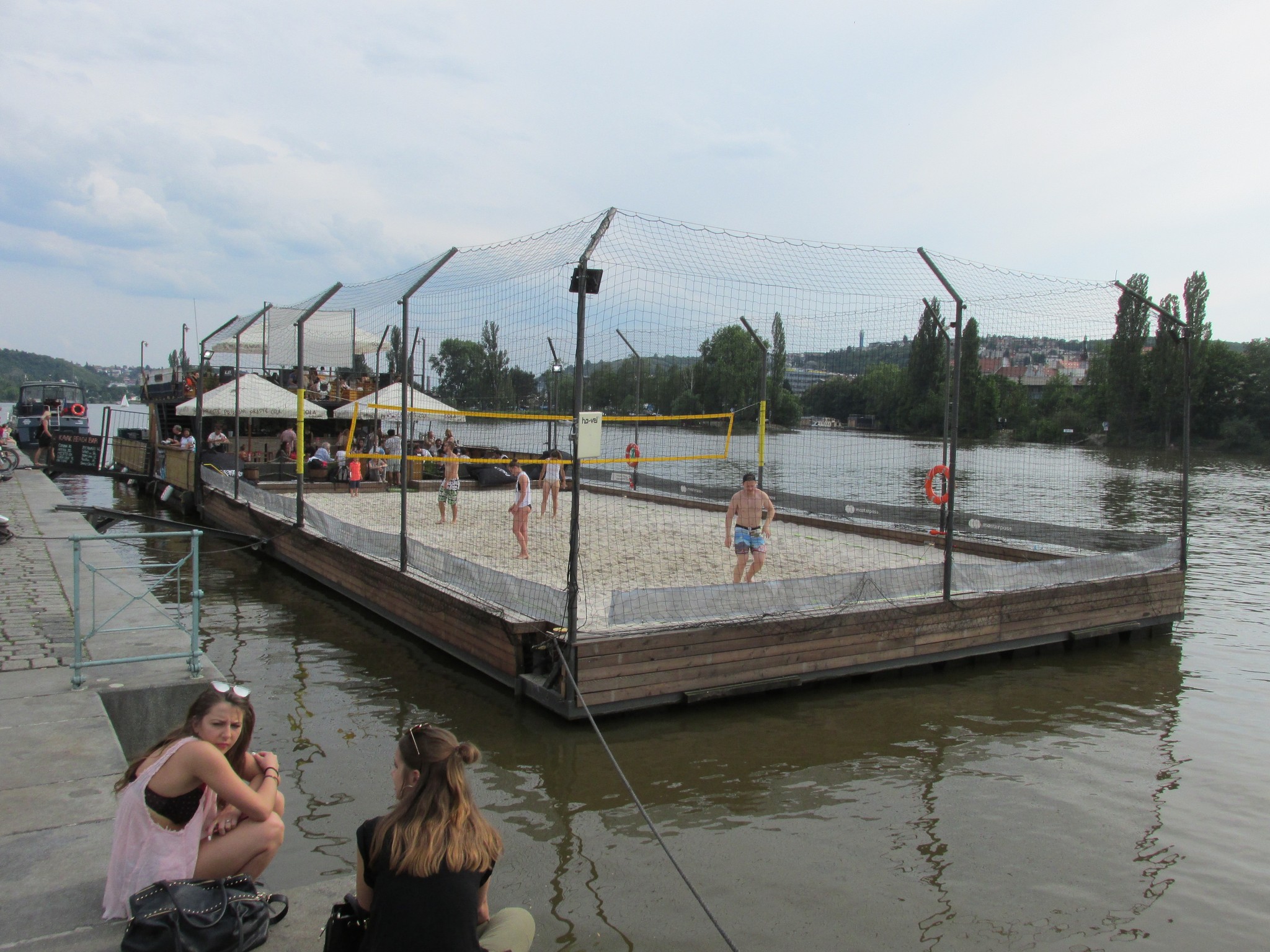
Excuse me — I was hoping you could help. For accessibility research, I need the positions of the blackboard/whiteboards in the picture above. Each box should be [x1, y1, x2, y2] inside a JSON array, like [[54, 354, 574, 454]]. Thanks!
[[53, 431, 102, 471]]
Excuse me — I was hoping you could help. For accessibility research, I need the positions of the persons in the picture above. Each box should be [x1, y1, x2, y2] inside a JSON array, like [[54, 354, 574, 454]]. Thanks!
[[435, 440, 460, 525], [537, 449, 566, 519], [368, 452, 388, 483], [349, 458, 363, 497], [413, 429, 509, 466], [276, 422, 389, 483], [238, 444, 248, 459], [207, 426, 229, 453], [287, 362, 410, 400], [102, 680, 285, 925], [0, 424, 17, 444], [166, 425, 196, 452], [508, 456, 532, 559], [725, 472, 775, 583], [355, 722, 535, 952], [191, 371, 200, 381], [34, 410, 55, 465]]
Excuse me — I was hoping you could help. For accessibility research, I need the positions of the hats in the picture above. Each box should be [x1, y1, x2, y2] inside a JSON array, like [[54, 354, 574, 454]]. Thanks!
[[743, 473, 756, 481]]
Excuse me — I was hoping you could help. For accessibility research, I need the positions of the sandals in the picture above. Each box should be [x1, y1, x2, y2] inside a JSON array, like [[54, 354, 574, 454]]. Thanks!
[[34, 462, 42, 465]]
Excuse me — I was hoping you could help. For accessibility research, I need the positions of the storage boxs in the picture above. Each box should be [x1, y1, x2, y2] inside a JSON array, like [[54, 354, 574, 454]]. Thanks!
[[329, 381, 375, 402]]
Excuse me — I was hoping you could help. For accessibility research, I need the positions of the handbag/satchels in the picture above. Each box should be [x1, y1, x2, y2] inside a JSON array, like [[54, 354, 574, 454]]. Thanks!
[[37, 419, 47, 441], [317, 893, 372, 952], [120, 873, 290, 952]]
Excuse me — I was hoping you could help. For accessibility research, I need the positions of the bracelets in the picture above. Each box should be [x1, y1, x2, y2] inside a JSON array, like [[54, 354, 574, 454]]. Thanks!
[[265, 766, 279, 776], [264, 774, 280, 785]]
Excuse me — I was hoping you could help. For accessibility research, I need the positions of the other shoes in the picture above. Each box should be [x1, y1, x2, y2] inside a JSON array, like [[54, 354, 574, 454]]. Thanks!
[[383, 480, 388, 483], [378, 479, 382, 483]]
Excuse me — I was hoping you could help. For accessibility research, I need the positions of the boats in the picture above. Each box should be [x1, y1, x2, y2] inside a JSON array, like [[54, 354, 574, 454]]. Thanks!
[[6, 379, 90, 448]]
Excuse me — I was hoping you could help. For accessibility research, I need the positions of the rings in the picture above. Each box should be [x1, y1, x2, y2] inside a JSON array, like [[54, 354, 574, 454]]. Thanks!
[[226, 819, 232, 823]]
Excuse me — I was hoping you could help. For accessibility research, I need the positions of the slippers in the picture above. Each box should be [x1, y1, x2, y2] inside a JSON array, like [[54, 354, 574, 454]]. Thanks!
[[398, 484, 402, 488]]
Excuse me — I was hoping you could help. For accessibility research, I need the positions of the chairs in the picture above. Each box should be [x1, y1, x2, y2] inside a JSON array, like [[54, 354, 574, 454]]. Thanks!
[[279, 369, 297, 394]]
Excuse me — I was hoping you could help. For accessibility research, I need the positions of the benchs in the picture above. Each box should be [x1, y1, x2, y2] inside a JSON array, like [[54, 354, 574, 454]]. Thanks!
[[244, 461, 339, 481]]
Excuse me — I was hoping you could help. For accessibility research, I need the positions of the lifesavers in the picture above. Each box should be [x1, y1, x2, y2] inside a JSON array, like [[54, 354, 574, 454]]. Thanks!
[[625, 443, 640, 467], [925, 465, 956, 505], [71, 404, 85, 415], [317, 380, 331, 396]]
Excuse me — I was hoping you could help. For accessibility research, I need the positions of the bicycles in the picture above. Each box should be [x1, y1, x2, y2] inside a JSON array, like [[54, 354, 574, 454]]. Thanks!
[[0, 443, 20, 474]]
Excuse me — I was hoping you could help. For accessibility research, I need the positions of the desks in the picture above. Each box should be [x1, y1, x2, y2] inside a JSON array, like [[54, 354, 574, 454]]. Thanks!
[[251, 455, 268, 462], [212, 441, 234, 453]]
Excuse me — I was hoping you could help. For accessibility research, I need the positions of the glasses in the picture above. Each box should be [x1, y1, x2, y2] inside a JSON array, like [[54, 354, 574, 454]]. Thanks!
[[445, 445, 446, 447], [408, 722, 433, 754], [204, 680, 251, 701]]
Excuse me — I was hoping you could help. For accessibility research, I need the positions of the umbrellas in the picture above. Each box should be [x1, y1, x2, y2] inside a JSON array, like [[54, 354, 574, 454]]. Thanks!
[[332, 382, 466, 436], [176, 373, 327, 462]]
